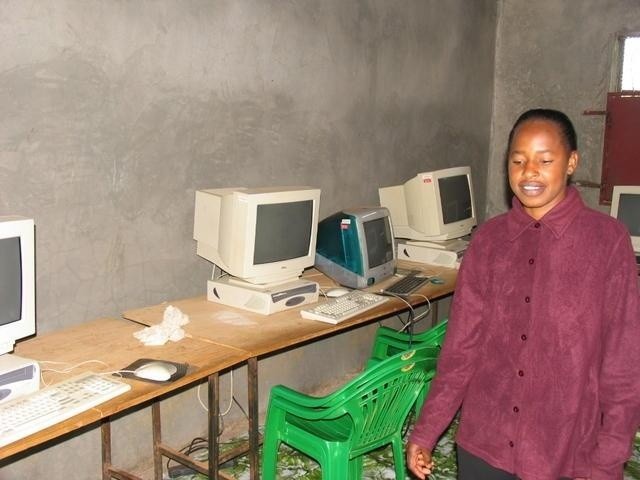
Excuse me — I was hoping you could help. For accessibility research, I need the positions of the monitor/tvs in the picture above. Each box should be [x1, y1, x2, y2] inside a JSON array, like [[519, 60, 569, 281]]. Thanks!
[[611, 185, 640, 255], [315, 207, 395, 290], [0, 217, 38, 352], [377, 166, 478, 242], [193, 187, 321, 284]]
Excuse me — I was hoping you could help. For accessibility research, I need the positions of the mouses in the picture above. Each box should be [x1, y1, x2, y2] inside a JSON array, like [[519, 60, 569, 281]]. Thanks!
[[135, 362, 178, 381], [327, 288, 348, 297]]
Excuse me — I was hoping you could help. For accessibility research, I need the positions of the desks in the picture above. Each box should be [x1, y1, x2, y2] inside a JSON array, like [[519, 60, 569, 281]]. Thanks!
[[124, 259, 459, 478], [1, 314, 253, 479]]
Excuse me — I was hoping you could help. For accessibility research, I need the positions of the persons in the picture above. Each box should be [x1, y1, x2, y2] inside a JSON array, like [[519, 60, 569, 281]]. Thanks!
[[405, 108, 639, 479]]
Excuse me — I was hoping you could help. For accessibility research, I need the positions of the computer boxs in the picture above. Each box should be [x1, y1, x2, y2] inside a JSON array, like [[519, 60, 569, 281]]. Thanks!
[[206, 280, 318, 316], [398, 244, 466, 268], [1, 356, 40, 399]]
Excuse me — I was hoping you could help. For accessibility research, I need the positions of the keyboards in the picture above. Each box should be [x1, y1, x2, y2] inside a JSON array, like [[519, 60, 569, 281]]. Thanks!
[[300, 291, 389, 326], [0, 372, 131, 445], [386, 277, 429, 297]]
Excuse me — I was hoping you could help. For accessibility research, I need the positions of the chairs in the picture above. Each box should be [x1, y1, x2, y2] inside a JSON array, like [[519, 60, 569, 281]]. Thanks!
[[262, 319, 448, 479]]
